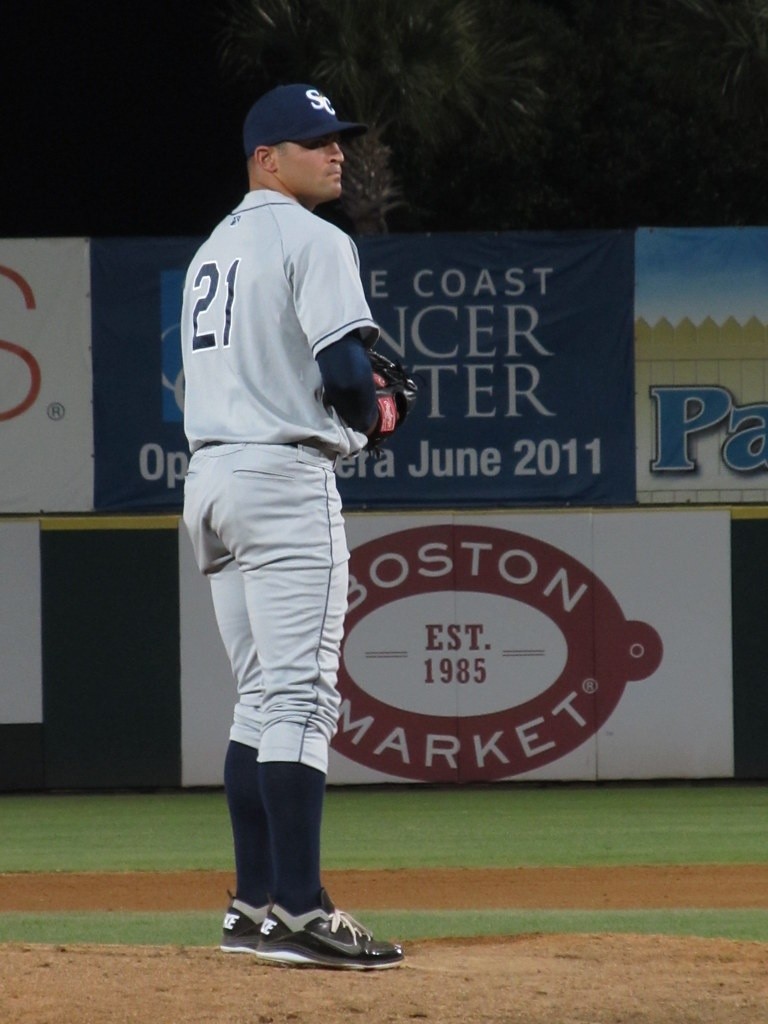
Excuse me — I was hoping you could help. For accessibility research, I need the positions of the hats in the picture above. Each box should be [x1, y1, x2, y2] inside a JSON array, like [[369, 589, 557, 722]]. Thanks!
[[243, 84, 369, 160]]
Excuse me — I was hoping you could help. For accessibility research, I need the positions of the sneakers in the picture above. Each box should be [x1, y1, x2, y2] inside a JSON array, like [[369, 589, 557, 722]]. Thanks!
[[254, 888, 405, 971], [219, 890, 272, 955]]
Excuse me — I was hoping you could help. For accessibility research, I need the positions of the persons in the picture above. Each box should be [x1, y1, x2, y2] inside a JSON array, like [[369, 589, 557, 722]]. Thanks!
[[180, 83, 419, 972]]
